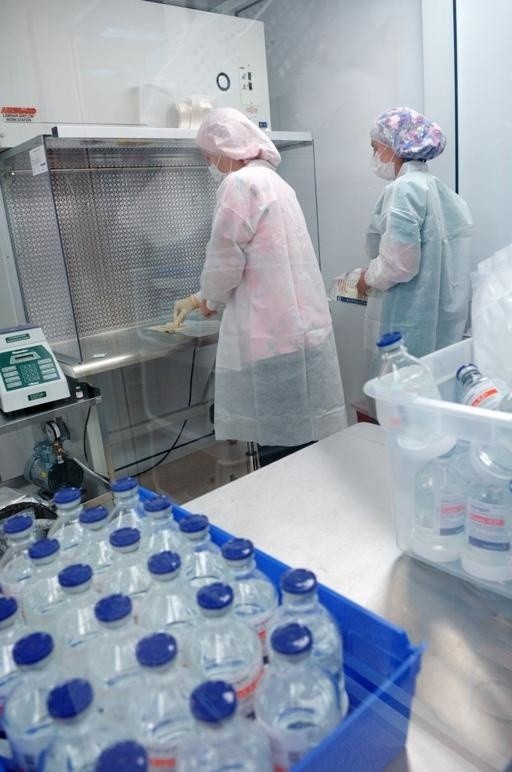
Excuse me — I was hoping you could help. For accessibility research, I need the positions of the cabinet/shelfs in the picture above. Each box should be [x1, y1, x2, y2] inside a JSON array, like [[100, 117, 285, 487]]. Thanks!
[[0, 118, 329, 510]]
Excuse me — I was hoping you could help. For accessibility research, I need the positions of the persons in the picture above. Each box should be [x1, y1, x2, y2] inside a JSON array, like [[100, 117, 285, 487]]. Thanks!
[[358, 106, 476, 422], [172, 106, 349, 445]]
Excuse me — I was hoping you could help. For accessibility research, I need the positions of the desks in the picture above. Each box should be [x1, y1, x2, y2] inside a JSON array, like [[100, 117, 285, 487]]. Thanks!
[[179, 415, 510, 770], [1, 372, 108, 500]]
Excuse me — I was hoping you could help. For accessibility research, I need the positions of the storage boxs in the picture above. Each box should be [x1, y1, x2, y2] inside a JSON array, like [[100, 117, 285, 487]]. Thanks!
[[1, 474, 425, 770], [359, 333, 510, 596]]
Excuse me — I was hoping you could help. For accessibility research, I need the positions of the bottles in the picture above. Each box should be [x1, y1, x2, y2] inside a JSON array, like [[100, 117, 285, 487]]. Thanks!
[[434, 364, 511, 480], [409, 449, 465, 561], [376, 332, 445, 451], [462, 480, 511, 582], [0, 477, 344, 771]]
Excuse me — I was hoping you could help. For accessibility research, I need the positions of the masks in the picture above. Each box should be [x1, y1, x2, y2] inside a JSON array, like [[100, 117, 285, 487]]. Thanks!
[[207, 156, 233, 183], [372, 149, 397, 181]]
